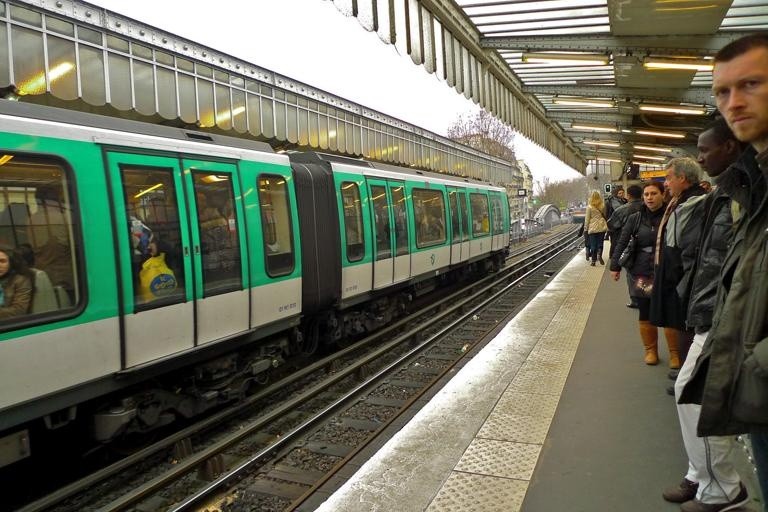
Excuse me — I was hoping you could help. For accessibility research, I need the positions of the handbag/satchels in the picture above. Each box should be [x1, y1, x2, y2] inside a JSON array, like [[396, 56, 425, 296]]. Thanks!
[[617, 234, 638, 268]]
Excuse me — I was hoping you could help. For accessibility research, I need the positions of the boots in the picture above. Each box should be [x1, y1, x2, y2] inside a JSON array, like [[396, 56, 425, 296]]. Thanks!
[[638, 321, 658, 365], [663, 328, 682, 369]]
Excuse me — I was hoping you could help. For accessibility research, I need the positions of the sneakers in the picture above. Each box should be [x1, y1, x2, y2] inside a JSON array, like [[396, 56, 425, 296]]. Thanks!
[[590, 262, 595, 266], [663, 477, 699, 502], [598, 256, 604, 265], [680, 481, 750, 512]]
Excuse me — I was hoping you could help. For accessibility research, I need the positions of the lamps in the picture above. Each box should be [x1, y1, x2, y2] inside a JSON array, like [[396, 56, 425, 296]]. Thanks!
[[512, 48, 717, 170]]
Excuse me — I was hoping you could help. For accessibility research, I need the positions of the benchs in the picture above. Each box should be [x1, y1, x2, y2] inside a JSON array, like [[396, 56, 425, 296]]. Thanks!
[[24, 264, 56, 319], [53, 285, 74, 308]]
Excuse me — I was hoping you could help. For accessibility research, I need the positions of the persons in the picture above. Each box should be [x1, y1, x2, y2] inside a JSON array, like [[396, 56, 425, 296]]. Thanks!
[[664, 120, 751, 510], [0, 189, 232, 326], [340, 182, 506, 261], [608, 182, 680, 368], [676, 33, 767, 511], [581, 179, 712, 268], [648, 158, 710, 394]]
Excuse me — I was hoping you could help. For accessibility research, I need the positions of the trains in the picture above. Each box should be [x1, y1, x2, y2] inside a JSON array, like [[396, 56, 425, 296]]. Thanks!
[[0, 112, 510, 489]]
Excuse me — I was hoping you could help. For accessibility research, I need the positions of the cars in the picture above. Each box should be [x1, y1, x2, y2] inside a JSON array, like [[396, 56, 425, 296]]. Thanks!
[[559, 208, 574, 217]]
